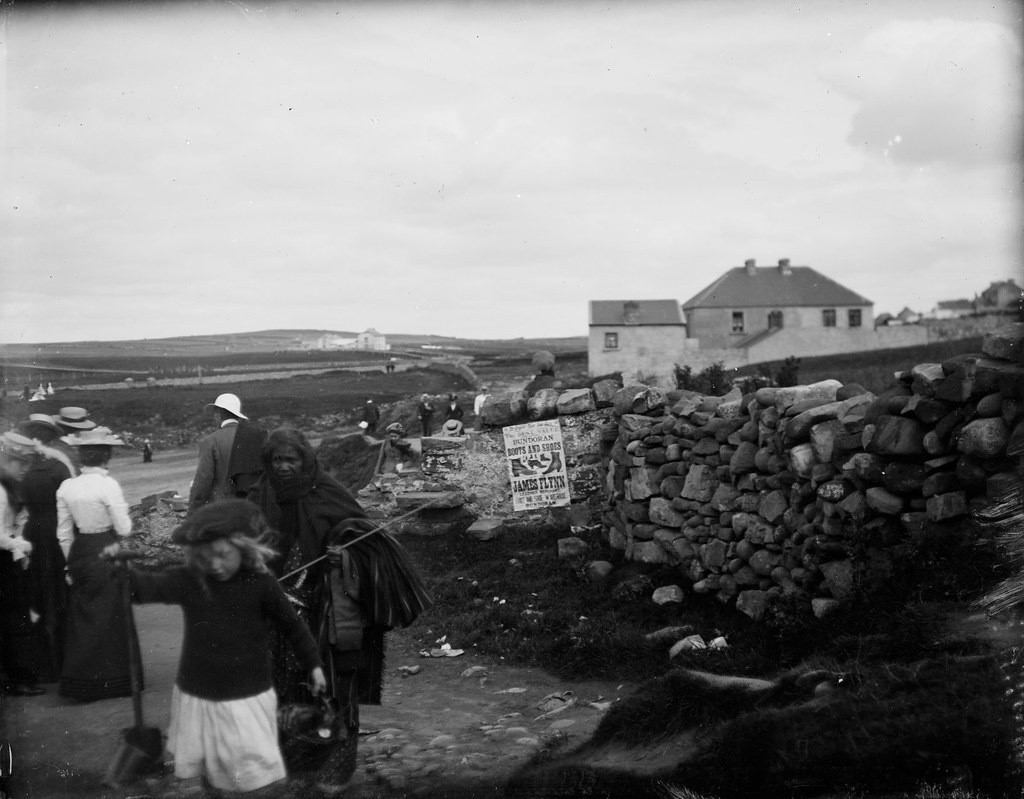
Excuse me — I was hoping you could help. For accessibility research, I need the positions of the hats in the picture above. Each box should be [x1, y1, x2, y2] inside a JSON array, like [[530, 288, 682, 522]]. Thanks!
[[443, 419, 462, 434], [18, 413, 64, 435], [60, 427, 125, 445], [204, 393, 249, 420], [172, 500, 258, 545], [51, 406, 97, 429], [0, 431, 36, 458]]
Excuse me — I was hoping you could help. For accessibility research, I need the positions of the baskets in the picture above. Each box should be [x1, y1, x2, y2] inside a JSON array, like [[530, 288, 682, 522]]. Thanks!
[[280, 682, 347, 771]]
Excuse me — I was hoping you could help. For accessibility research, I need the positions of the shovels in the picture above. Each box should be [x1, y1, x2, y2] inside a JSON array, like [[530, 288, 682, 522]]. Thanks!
[[105, 549, 164, 776]]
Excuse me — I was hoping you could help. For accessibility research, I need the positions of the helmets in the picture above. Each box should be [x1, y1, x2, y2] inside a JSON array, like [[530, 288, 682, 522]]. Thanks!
[[386, 422, 405, 433]]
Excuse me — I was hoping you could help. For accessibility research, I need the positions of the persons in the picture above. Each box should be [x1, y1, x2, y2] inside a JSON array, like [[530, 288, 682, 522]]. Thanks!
[[51, 406, 97, 476], [417, 394, 437, 436], [473, 385, 491, 416], [144, 439, 153, 463], [359, 399, 380, 436], [0, 432, 48, 697], [245, 427, 430, 785], [444, 394, 465, 435], [18, 411, 76, 683], [55, 430, 143, 701], [373, 423, 420, 477], [97, 500, 327, 799], [186, 393, 249, 518]]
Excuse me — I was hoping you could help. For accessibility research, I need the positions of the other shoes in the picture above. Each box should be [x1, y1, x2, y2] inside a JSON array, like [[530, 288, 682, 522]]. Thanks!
[[14, 683, 45, 696]]
